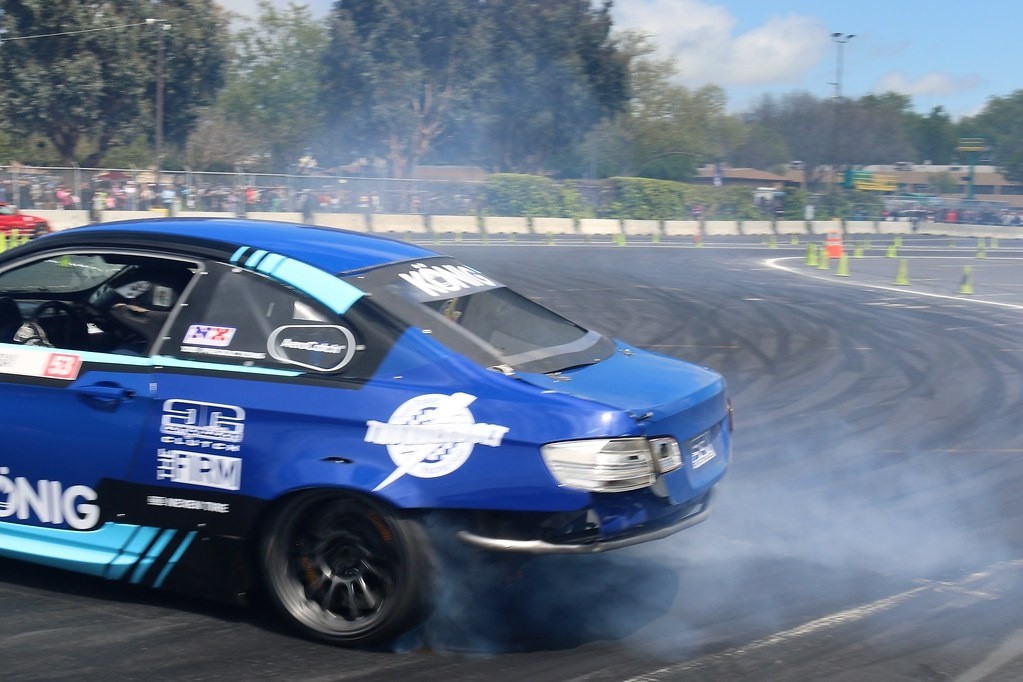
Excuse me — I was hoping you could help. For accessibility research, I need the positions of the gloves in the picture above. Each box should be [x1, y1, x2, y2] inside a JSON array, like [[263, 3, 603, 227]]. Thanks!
[[11, 319, 55, 348]]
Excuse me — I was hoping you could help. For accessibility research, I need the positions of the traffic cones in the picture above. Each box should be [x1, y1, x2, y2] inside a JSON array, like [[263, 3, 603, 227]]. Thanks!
[[851, 243, 864, 260], [803, 241, 818, 266], [693, 232, 702, 248], [767, 233, 779, 249], [833, 249, 852, 278], [884, 244, 897, 259], [974, 237, 998, 259], [894, 236, 903, 246], [891, 257, 912, 286], [403, 231, 628, 247], [788, 230, 800, 246], [955, 264, 975, 294], [650, 229, 660, 244], [816, 242, 830, 270], [862, 237, 872, 251]]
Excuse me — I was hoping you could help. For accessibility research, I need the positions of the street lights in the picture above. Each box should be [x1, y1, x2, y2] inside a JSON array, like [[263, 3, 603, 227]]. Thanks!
[[829, 33, 857, 215]]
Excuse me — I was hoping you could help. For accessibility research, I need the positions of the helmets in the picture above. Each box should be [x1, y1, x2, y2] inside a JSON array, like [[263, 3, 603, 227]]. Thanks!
[[88, 262, 193, 348]]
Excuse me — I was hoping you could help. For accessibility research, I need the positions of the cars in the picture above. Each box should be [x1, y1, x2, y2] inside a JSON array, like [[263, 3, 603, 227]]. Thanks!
[[1, 199, 736, 653]]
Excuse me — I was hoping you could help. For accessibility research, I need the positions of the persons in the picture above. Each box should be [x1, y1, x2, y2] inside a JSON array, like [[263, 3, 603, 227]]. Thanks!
[[692, 203, 703, 220], [881, 207, 891, 221], [13, 260, 201, 358], [856, 206, 869, 220], [890, 202, 1023, 227], [409, 197, 421, 214], [0, 181, 381, 213]]
